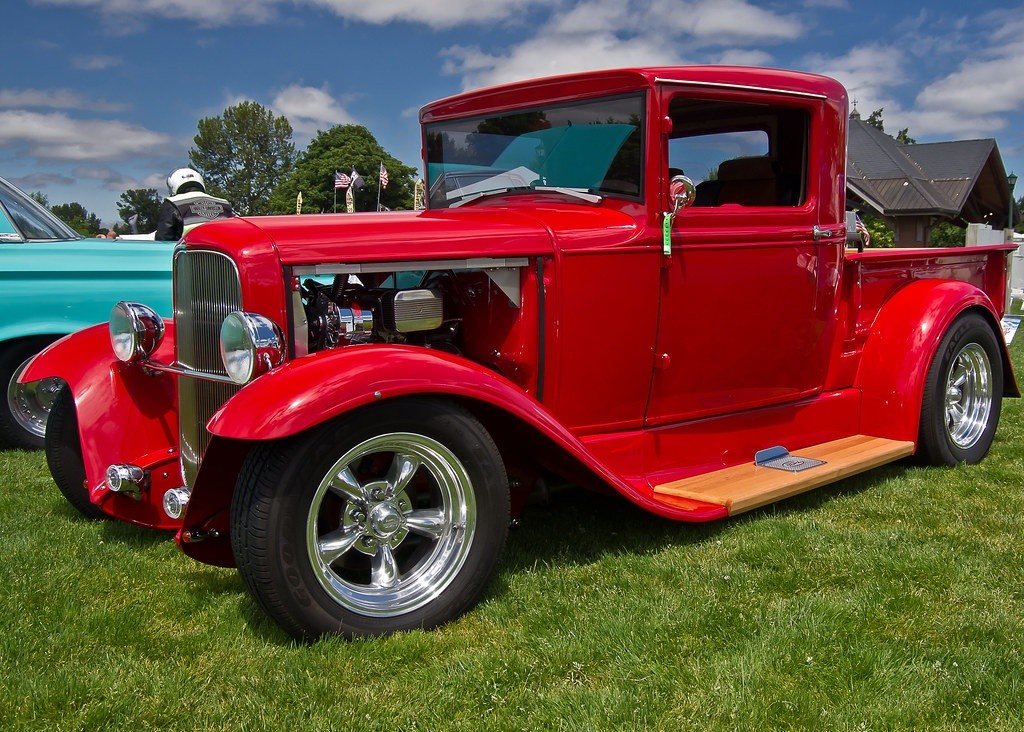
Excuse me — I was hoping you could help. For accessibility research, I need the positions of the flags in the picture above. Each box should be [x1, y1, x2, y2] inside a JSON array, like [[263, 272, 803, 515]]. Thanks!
[[380, 162, 388, 189], [351, 165, 365, 188], [335, 170, 350, 188]]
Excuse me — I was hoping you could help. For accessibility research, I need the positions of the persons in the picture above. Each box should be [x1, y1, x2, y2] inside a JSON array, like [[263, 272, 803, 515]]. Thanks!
[[96, 231, 116, 239], [154, 168, 232, 241]]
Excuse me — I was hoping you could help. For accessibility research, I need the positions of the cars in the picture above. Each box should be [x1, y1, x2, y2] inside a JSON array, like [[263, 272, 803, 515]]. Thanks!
[[0, 174, 386, 453]]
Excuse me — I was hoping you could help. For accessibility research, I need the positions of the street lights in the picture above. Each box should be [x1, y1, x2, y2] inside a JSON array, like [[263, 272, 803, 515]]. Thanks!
[[1005, 170, 1018, 228]]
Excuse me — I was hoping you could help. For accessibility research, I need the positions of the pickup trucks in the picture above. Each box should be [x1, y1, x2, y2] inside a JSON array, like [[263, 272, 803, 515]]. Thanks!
[[16, 66, 1021, 643]]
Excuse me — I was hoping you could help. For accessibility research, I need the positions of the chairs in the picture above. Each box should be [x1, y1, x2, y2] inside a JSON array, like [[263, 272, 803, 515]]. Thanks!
[[692, 155, 792, 210]]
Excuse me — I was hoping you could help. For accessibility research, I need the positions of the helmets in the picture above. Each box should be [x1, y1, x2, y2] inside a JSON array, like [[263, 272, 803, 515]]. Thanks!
[[166, 167, 207, 195]]
